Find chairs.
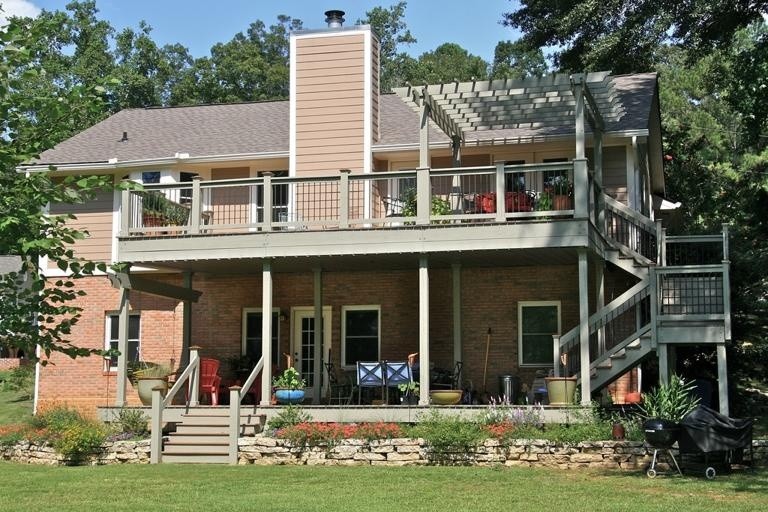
[324,361,464,404]
[184,356,223,405]
[381,196,413,230]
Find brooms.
[475,327,492,404]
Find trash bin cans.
[498,374,520,405]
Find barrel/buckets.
[201,357,221,390]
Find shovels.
[480,327,492,405]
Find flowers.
[543,175,574,200]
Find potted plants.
[271,367,307,405]
[141,191,189,237]
[133,361,173,407]
[630,372,703,448]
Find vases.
[552,195,572,210]
[544,376,577,405]
